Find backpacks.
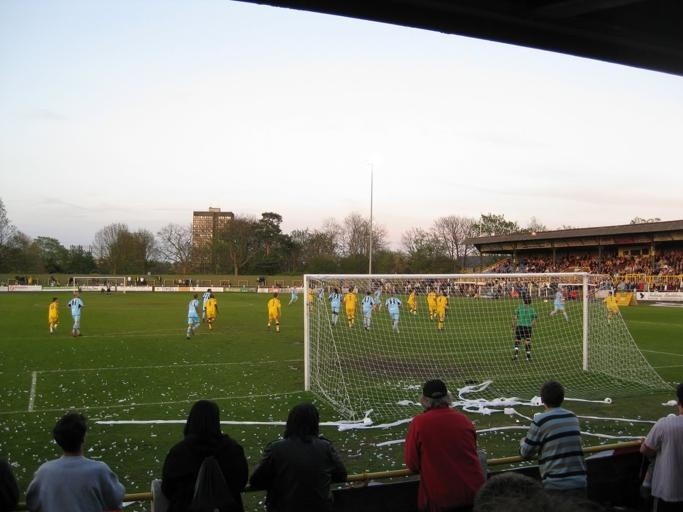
[188,436,231,512]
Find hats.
[423,380,447,399]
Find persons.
[250,402,347,512]
[265,293,281,333]
[25,414,127,512]
[204,294,218,330]
[492,249,683,292]
[0,459,19,512]
[548,288,568,321]
[640,382,683,511]
[519,381,588,498]
[202,289,214,322]
[0,275,284,297]
[403,379,487,512]
[161,399,248,512]
[552,494,606,512]
[48,297,60,334]
[604,291,619,326]
[185,294,201,339]
[291,277,451,335]
[473,472,555,512]
[66,292,86,337]
[512,295,538,363]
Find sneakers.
[510,354,532,361]
